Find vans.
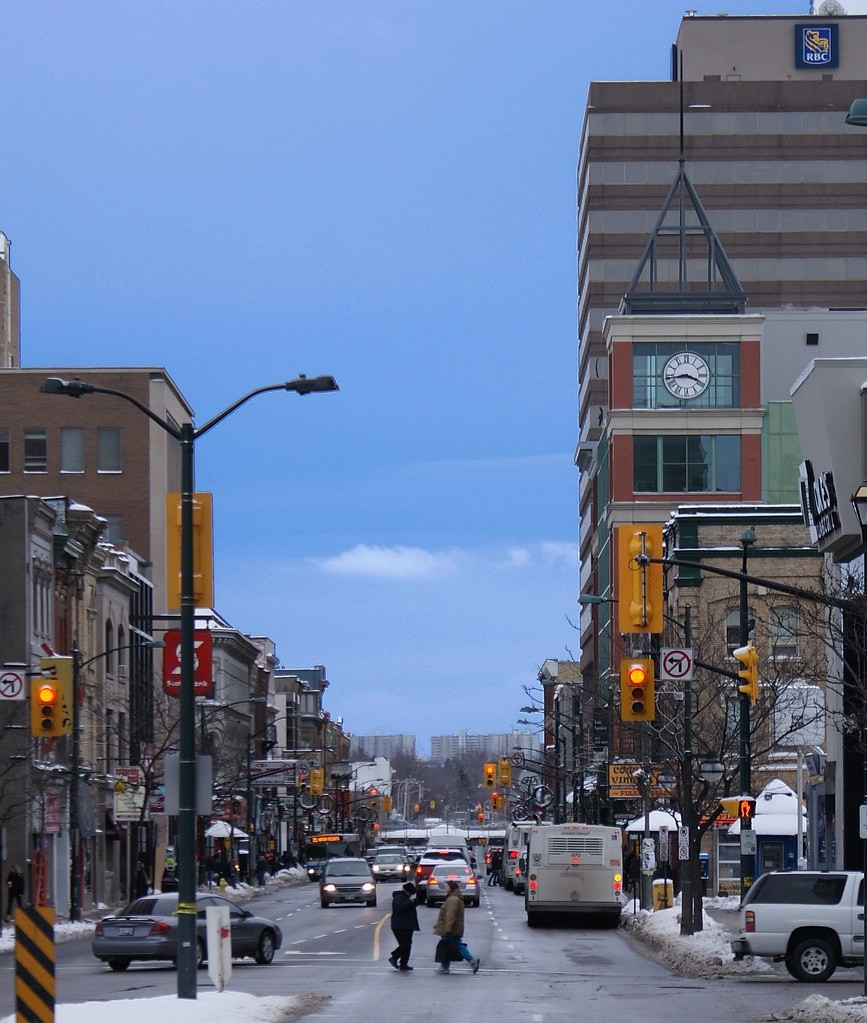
[483,822,553,893]
[731,869,865,982]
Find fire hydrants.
[219,878,227,893]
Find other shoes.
[628,886,632,893]
[389,957,399,969]
[493,884,497,886]
[399,964,413,971]
[471,958,480,974]
[437,966,450,975]
[488,884,492,886]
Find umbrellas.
[204,820,249,838]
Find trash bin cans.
[653,880,672,911]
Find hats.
[403,883,417,895]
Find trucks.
[519,824,623,928]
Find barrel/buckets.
[652,884,674,912]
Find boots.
[5,914,11,923]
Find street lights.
[520,706,585,823]
[336,764,377,835]
[513,745,561,826]
[312,759,353,836]
[293,745,338,862]
[247,714,302,884]
[517,719,567,825]
[198,697,265,886]
[578,594,725,935]
[540,679,623,825]
[354,778,383,852]
[70,641,165,920]
[361,778,430,830]
[39,373,340,999]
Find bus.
[302,834,358,883]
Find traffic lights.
[720,797,757,819]
[490,791,499,812]
[732,641,759,707]
[29,678,64,737]
[483,763,497,789]
[620,659,656,721]
[478,805,483,824]
[371,801,376,811]
[299,773,306,790]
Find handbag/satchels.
[435,940,467,962]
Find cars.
[415,837,483,908]
[316,858,378,907]
[92,892,282,971]
[364,846,426,883]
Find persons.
[388,882,421,971]
[433,880,481,973]
[4,863,25,924]
[622,847,640,894]
[256,850,297,886]
